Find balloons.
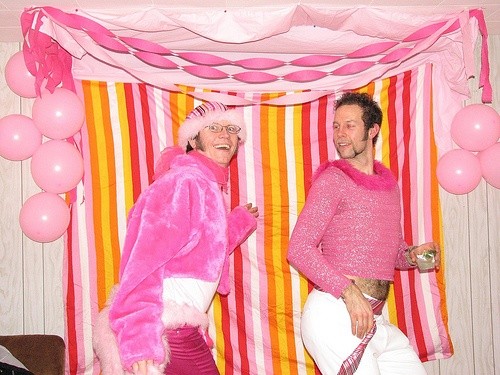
[449,104,500,152]
[30,139,84,194]
[19,193,70,243]
[5,50,53,99]
[0,114,42,161]
[437,149,482,195]
[32,88,85,140]
[478,142,500,189]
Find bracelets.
[406,246,419,266]
[341,279,355,299]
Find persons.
[287,93,442,375]
[93,102,259,375]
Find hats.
[178,101,247,150]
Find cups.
[414,249,435,274]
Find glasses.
[203,123,241,134]
